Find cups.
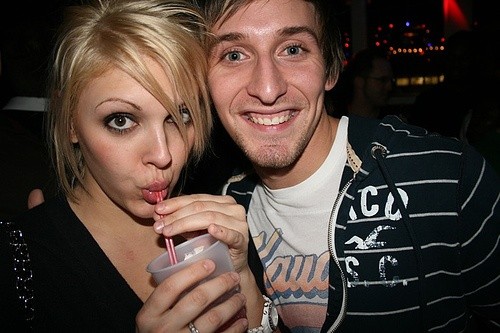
[145,234,241,312]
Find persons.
[28,0,500,333]
[0,0,280,333]
[340,51,395,118]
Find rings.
[186,321,203,333]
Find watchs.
[245,294,280,333]
